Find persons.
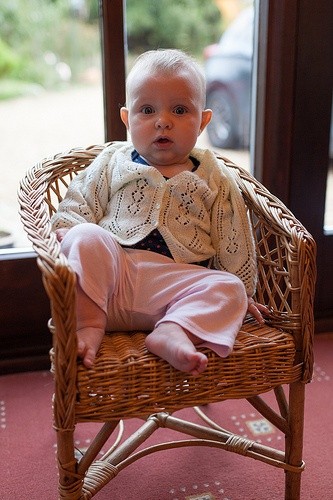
[50,50,271,376]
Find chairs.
[18,140,317,500]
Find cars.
[200,4,255,147]
